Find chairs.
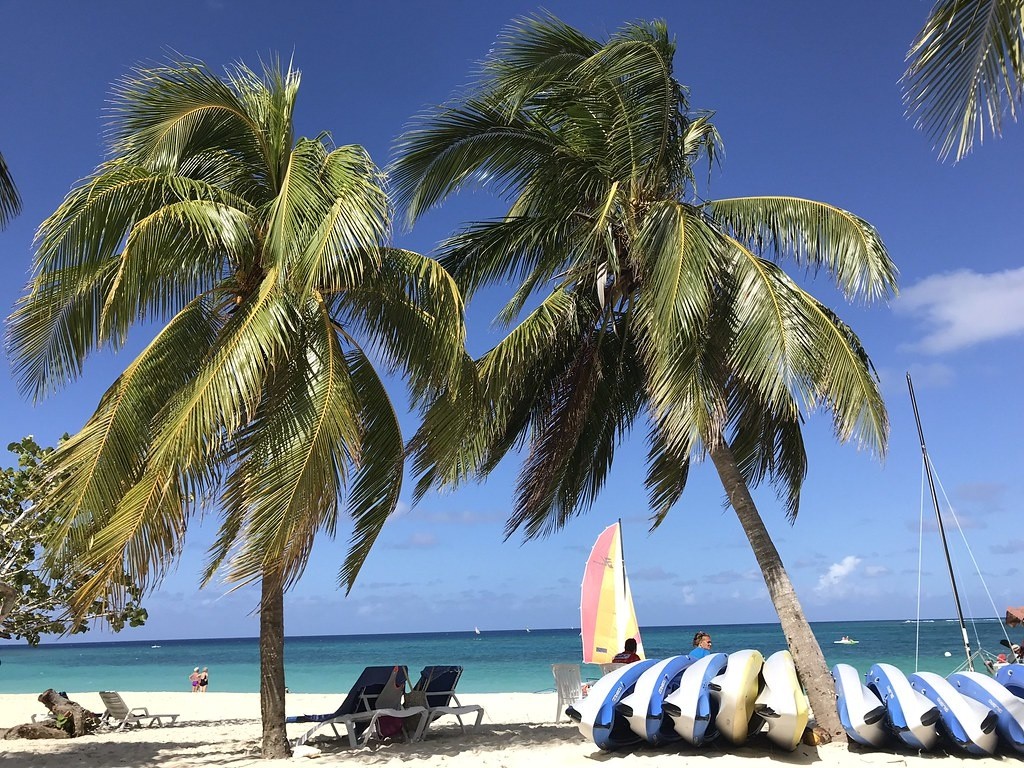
[551,664,585,723]
[89,692,180,732]
[598,662,628,676]
[286,666,484,751]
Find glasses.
[700,631,709,635]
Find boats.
[562,655,1024,758]
[151,645,161,648]
[833,640,861,645]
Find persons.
[197,667,209,692]
[689,631,711,658]
[842,636,849,641]
[189,667,200,692]
[1007,644,1024,664]
[612,638,641,663]
